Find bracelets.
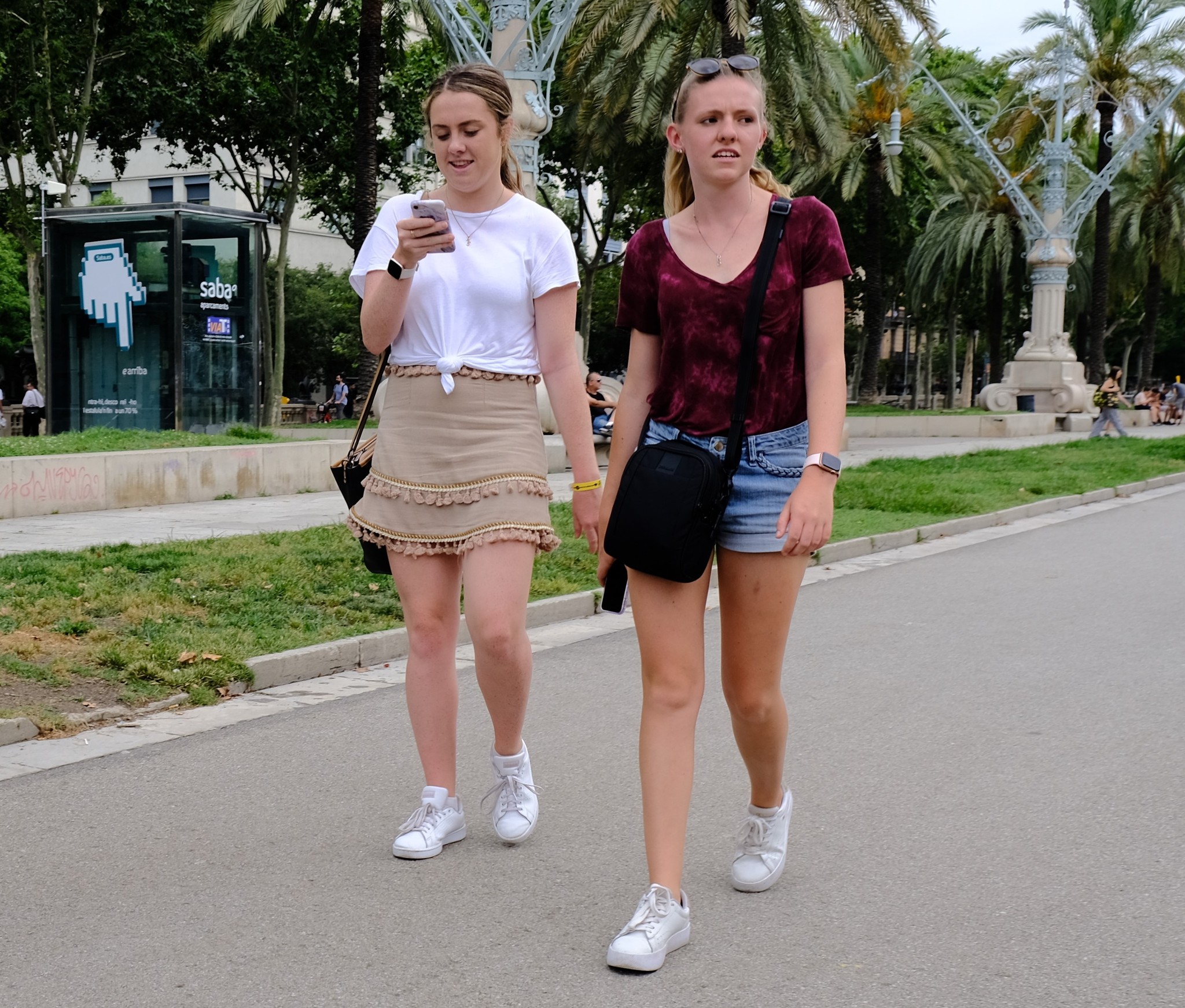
[569,479,601,492]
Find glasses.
[672,51,760,122]
[592,378,602,382]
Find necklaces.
[694,182,752,268]
[444,183,504,245]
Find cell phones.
[600,562,629,614]
[411,200,456,253]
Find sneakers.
[606,881,690,971]
[392,786,465,859]
[480,739,545,844]
[730,781,792,892]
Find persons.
[597,58,857,973]
[0,382,43,437]
[586,371,616,438]
[328,373,358,421]
[347,66,607,864]
[1087,366,1185,437]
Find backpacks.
[335,383,351,400]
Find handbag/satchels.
[26,409,41,424]
[330,344,392,574]
[603,440,728,584]
[1093,392,1120,409]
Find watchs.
[803,452,841,479]
[388,254,419,281]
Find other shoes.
[1152,418,1180,426]
[599,423,613,435]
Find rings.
[410,230,417,238]
